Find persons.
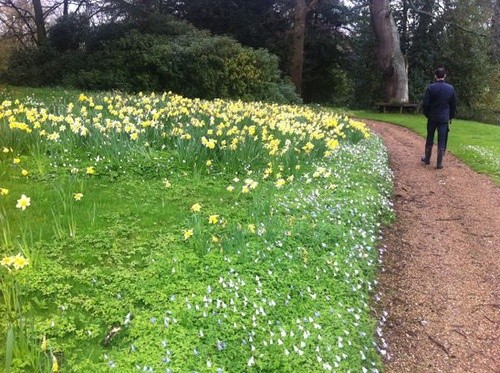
[419,66,458,168]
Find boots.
[420,144,433,165]
[436,145,443,168]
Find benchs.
[374,101,420,115]
